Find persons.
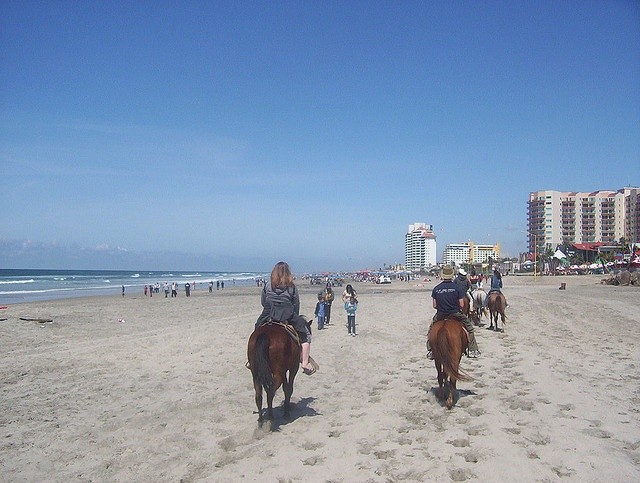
[313,294,327,330]
[163,282,170,298]
[171,282,178,297]
[320,281,334,325]
[184,282,191,297]
[208,280,213,293]
[450,268,474,314]
[219,273,417,291]
[192,280,196,289]
[482,269,510,310]
[424,263,482,359]
[468,269,480,291]
[343,296,357,337]
[342,284,359,303]
[142,280,160,297]
[253,259,315,372]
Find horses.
[427,318,478,409]
[486,290,509,330]
[471,288,488,322]
[247,315,313,422]
[462,295,469,317]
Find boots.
[564,282,566,289]
[559,282,564,289]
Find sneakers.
[352,332,356,336]
[426,349,433,359]
[468,349,481,357]
[348,332,351,335]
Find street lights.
[526,231,537,280]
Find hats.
[325,282,331,288]
[438,264,455,279]
[457,268,468,275]
